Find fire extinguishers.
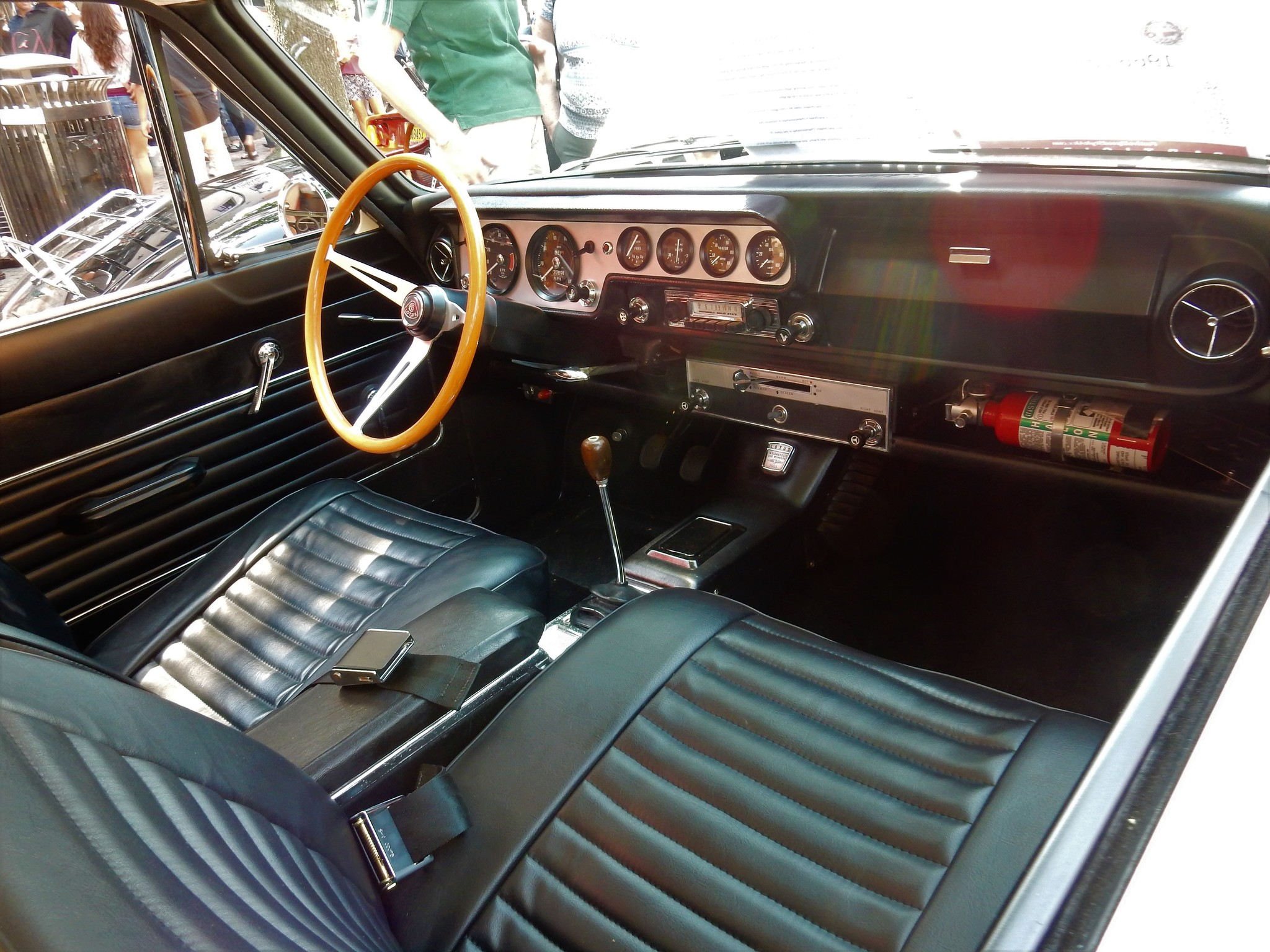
[942,380,1172,474]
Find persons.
[529,0,609,164]
[0,0,276,195]
[334,0,388,136]
[359,0,557,189]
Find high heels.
[244,144,259,161]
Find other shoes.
[227,141,243,152]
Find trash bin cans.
[0,74,144,266]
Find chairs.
[0,477,1107,952]
